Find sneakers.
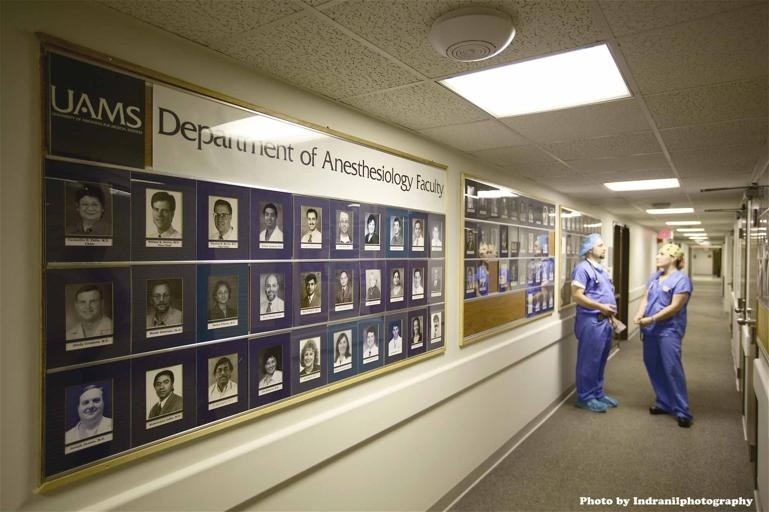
[574,396,608,413]
[596,394,619,407]
[649,405,673,413]
[676,409,691,427]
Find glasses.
[214,213,231,220]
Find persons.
[570,233,619,413]
[209,357,238,401]
[301,340,320,376]
[206,197,238,244]
[413,220,423,247]
[412,269,424,295]
[391,218,402,245]
[367,271,380,300]
[148,186,180,245]
[337,211,351,245]
[431,224,442,247]
[301,274,320,309]
[365,214,378,244]
[209,281,236,320]
[66,383,113,442]
[64,182,115,243]
[411,318,423,342]
[391,271,403,297]
[464,181,555,315]
[147,279,181,328]
[253,203,285,243]
[257,351,282,385]
[432,314,441,339]
[299,210,322,247]
[633,243,694,427]
[260,273,284,313]
[431,269,441,293]
[334,333,352,366]
[363,327,379,359]
[148,369,184,417]
[335,271,352,303]
[67,285,111,338]
[388,323,402,354]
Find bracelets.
[650,315,656,324]
